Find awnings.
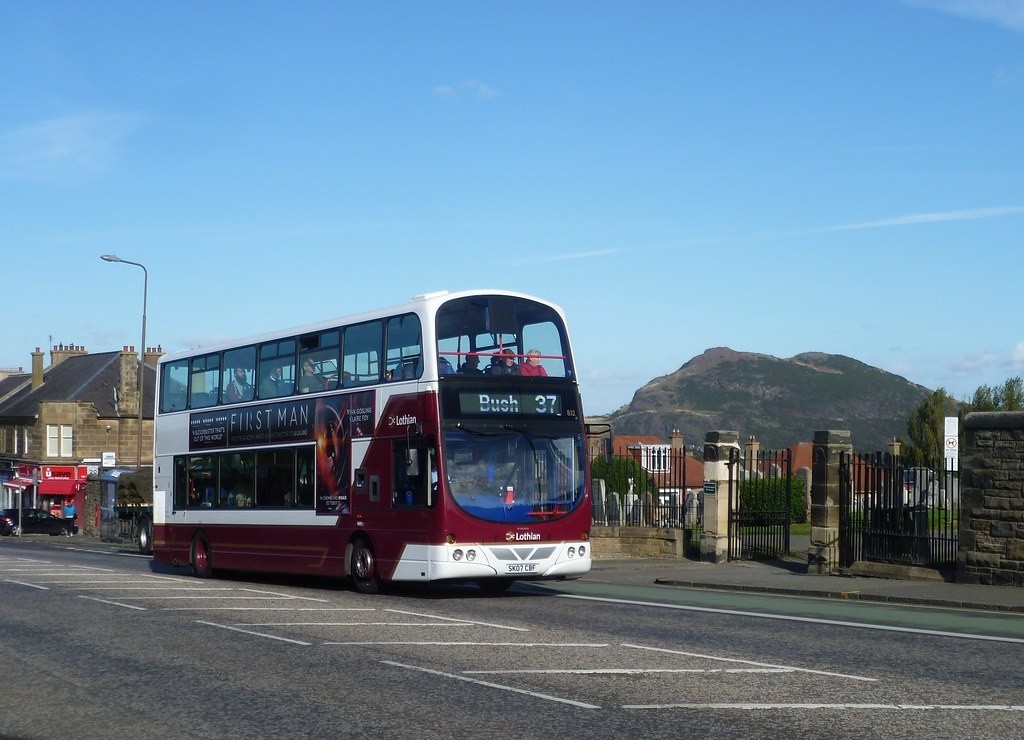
[2,477,39,492]
[39,478,77,495]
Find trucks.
[113,464,154,555]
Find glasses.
[304,364,317,367]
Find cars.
[0,508,79,538]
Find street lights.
[98,254,149,467]
[104,425,112,454]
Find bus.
[151,292,612,594]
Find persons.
[64,498,77,537]
[176,349,550,510]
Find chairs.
[193,477,228,507]
[183,372,356,406]
[439,359,491,375]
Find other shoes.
[67,535,69,538]
[70,533,73,538]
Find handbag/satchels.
[73,514,78,520]
